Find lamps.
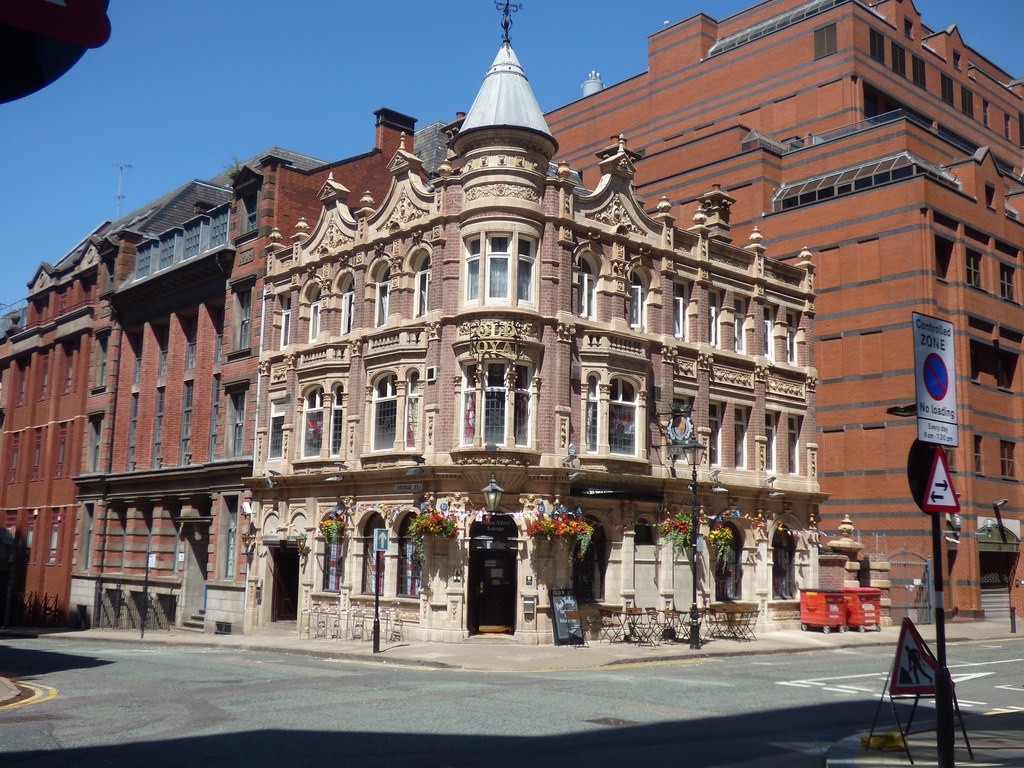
[406,468,423,475]
[325,476,343,481]
[769,493,785,498]
[334,462,348,472]
[708,470,721,482]
[942,516,965,536]
[993,498,1009,507]
[974,519,996,538]
[411,455,426,466]
[268,470,281,477]
[567,472,586,481]
[562,455,577,468]
[264,478,277,489]
[712,485,728,493]
[481,474,505,513]
[764,477,776,488]
[240,532,254,560]
[296,534,308,556]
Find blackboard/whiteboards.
[548,588,584,646]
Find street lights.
[681,430,708,651]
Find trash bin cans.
[837,587,881,633]
[798,588,850,634]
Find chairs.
[564,603,760,646]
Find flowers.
[707,523,733,575]
[408,509,459,567]
[525,512,594,569]
[659,510,693,564]
[319,519,347,546]
